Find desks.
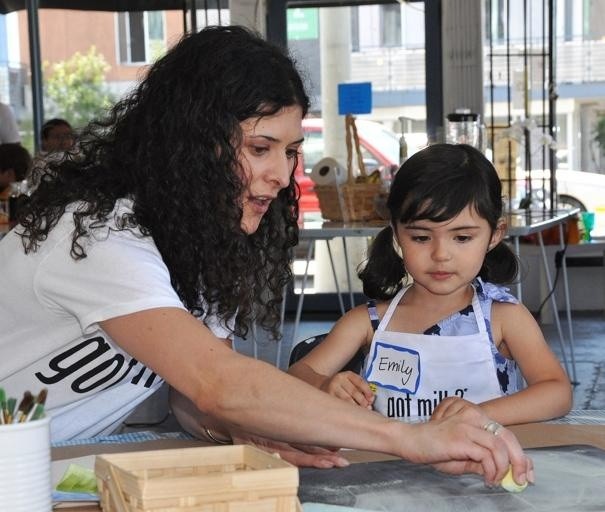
[278,206,582,392]
[50,406,605,511]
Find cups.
[0,410,52,512]
[446,112,487,157]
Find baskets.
[314,112,384,222]
[95,444,300,511]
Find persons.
[0,24,535,489]
[31,118,78,185]
[288,141,573,426]
[0,103,20,145]
[0,144,30,232]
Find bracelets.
[203,418,231,445]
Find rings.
[484,421,504,435]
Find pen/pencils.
[0,386,47,424]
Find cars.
[291,118,420,213]
